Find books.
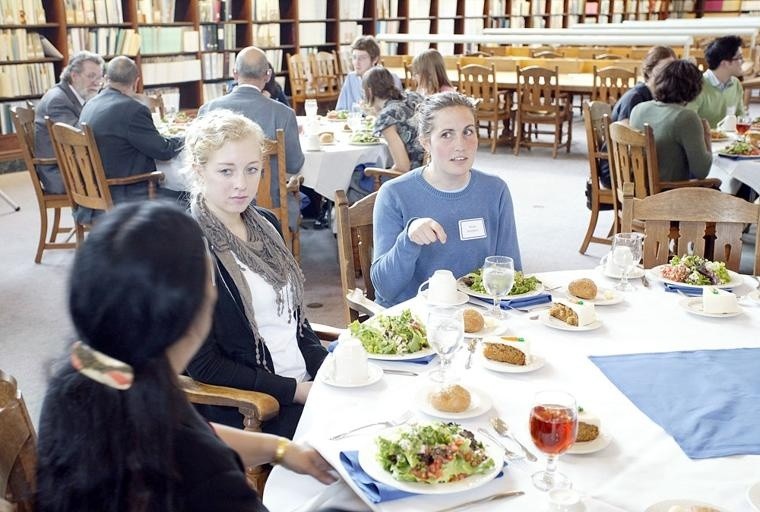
[64,0,329,117]
[338,1,679,56]
[0,0,57,135]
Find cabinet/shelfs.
[298,0,338,98]
[338,1,510,73]
[252,0,298,108]
[508,0,695,27]
[0,0,70,161]
[695,0,760,18]
[130,0,203,120]
[196,0,253,111]
[61,0,141,96]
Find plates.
[417,387,492,419]
[566,289,624,304]
[687,299,747,319]
[350,139,383,145]
[344,129,352,132]
[359,426,506,493]
[456,275,545,300]
[566,412,611,455]
[365,342,437,360]
[320,362,382,385]
[651,265,742,289]
[596,266,645,280]
[539,310,602,333]
[326,115,351,121]
[320,142,336,145]
[416,292,472,308]
[482,355,546,374]
[710,118,760,158]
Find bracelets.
[269,435,289,467]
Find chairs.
[0,370,39,512]
[166,310,348,504]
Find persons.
[33,49,106,197]
[27,198,339,512]
[597,34,758,262]
[410,48,456,99]
[173,107,327,472]
[345,65,426,203]
[71,55,191,231]
[369,90,525,311]
[333,34,405,115]
[194,44,306,236]
[226,57,292,108]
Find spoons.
[489,414,539,463]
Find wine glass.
[482,256,513,324]
[304,100,318,128]
[612,232,642,293]
[162,104,177,127]
[425,303,465,385]
[530,392,578,493]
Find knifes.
[437,492,525,510]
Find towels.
[339,450,507,503]
[325,340,437,365]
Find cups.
[304,134,322,149]
[419,268,457,303]
[331,338,367,379]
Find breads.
[430,382,472,414]
[567,277,598,302]
[549,299,581,329]
[460,307,485,333]
[574,421,599,443]
[482,339,526,368]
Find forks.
[478,428,527,466]
[330,410,413,441]
